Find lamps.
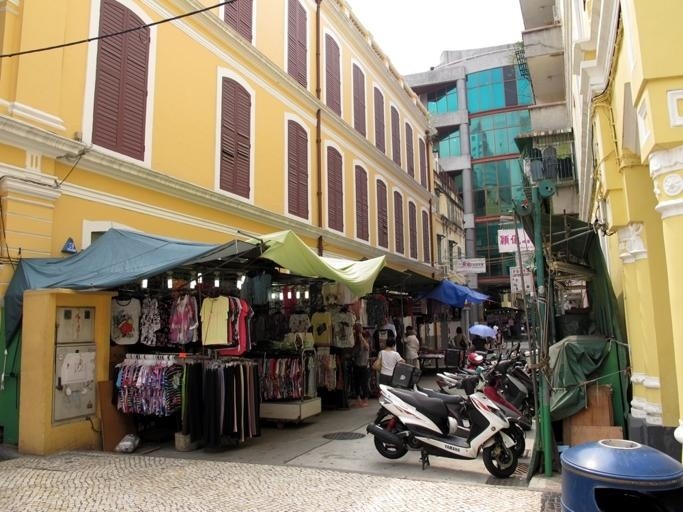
[592,198,608,237]
[140,271,247,290]
[271,283,311,301]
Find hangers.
[114,353,240,369]
[255,307,353,315]
[120,289,248,298]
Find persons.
[494,329,502,349]
[400,327,420,370]
[472,334,487,352]
[372,334,407,398]
[348,328,372,409]
[452,326,469,361]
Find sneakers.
[350,399,370,408]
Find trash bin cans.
[560,439,683,512]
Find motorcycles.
[366,341,539,479]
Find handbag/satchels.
[460,339,471,348]
[371,358,382,371]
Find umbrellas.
[468,323,496,340]
